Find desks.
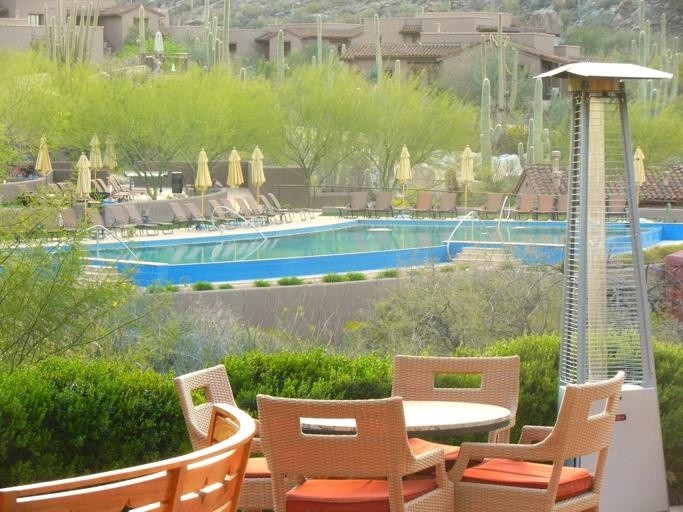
[247,400,512,450]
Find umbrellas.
[633,146,646,207]
[154,29,164,52]
[89,133,103,192]
[251,145,266,204]
[396,144,412,215]
[461,145,474,213]
[104,135,118,193]
[195,148,213,216]
[74,152,91,224]
[227,147,244,211]
[35,133,52,193]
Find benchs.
[0,399,258,511]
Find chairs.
[256,391,454,512]
[474,193,504,220]
[172,364,293,510]
[407,192,434,219]
[512,194,538,220]
[389,352,521,461]
[1,172,301,259]
[338,192,369,219]
[363,192,393,219]
[605,195,628,222]
[430,192,458,219]
[533,194,556,219]
[554,195,567,220]
[440,368,627,512]
